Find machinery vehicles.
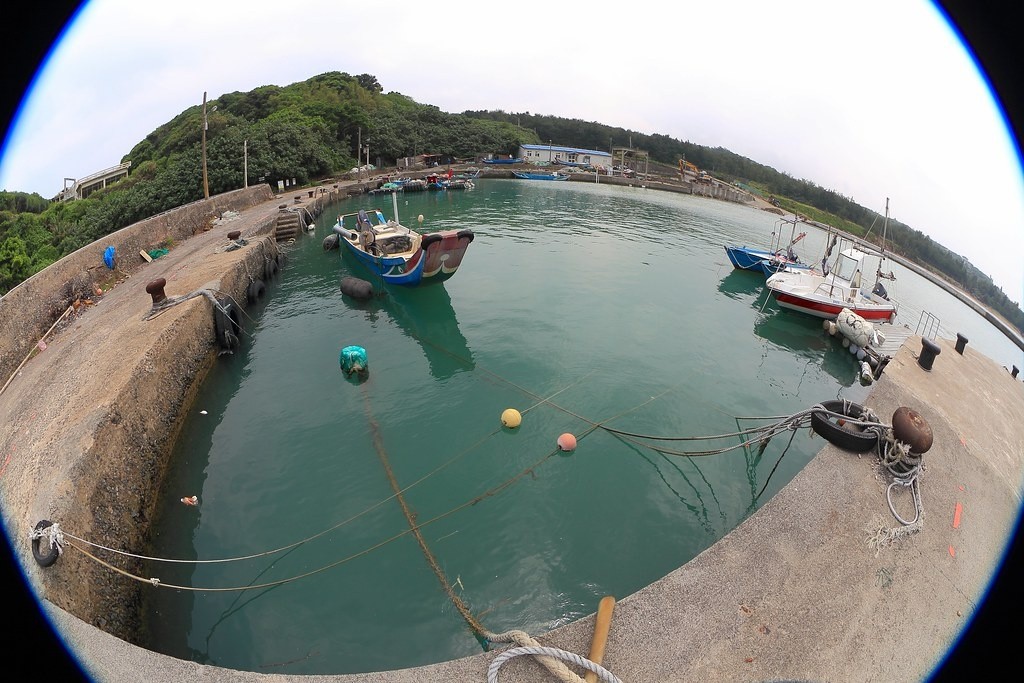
[679,158,713,186]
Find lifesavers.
[810,397,885,454]
[247,253,284,305]
[30,519,62,568]
[212,298,246,355]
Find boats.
[404,178,476,190]
[330,208,474,289]
[511,169,572,181]
[724,197,900,325]
